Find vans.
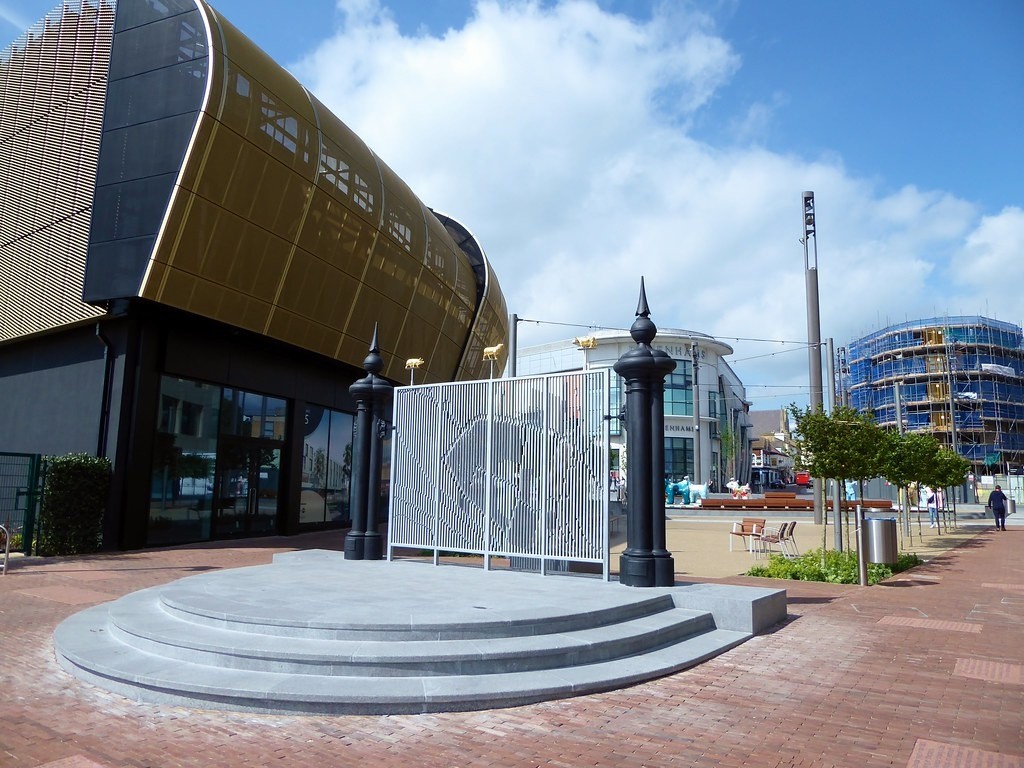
[177,452,247,504]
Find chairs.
[765,522,800,558]
[608,511,620,535]
[730,517,767,555]
[754,522,790,560]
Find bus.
[796,472,810,486]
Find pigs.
[405,356,424,369]
[572,336,597,348]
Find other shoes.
[930,524,935,528]
[1001,528,1007,531]
[936,524,939,527]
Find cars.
[770,479,786,489]
[806,480,813,488]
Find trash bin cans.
[861,517,898,564]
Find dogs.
[482,343,505,360]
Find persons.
[926,488,940,528]
[988,485,1008,531]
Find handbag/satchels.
[928,494,934,503]
